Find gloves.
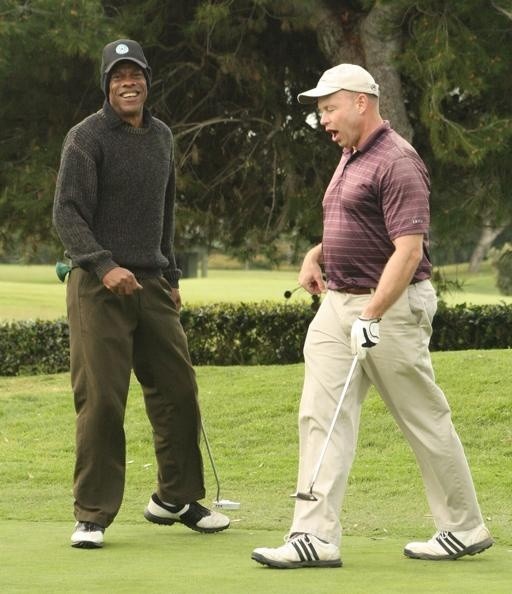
[350,316,381,361]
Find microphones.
[283,284,303,299]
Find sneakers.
[144,494,230,533]
[403,523,492,561]
[70,520,105,549]
[251,533,343,569]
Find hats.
[297,64,380,106]
[102,39,148,75]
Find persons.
[252,63,492,570]
[52,39,231,549]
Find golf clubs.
[200,419,240,508]
[290,356,358,502]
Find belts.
[342,284,377,295]
